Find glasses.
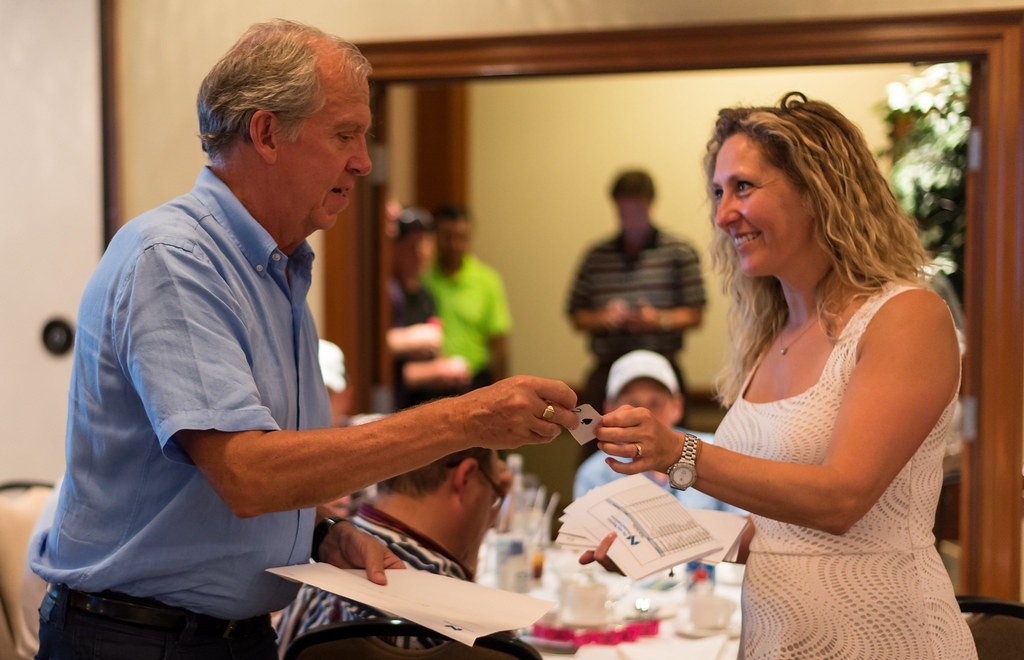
[444,456,508,511]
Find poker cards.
[563,402,608,448]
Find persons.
[275,380,514,660]
[564,170,705,458]
[387,201,512,413]
[28,18,580,660]
[573,92,979,660]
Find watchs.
[666,433,698,491]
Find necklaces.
[779,315,820,357]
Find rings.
[635,444,643,459]
[542,405,555,418]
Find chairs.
[0,483,57,659]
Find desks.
[479,543,747,660]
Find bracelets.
[309,517,351,559]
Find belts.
[49,584,272,641]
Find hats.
[606,350,679,403]
